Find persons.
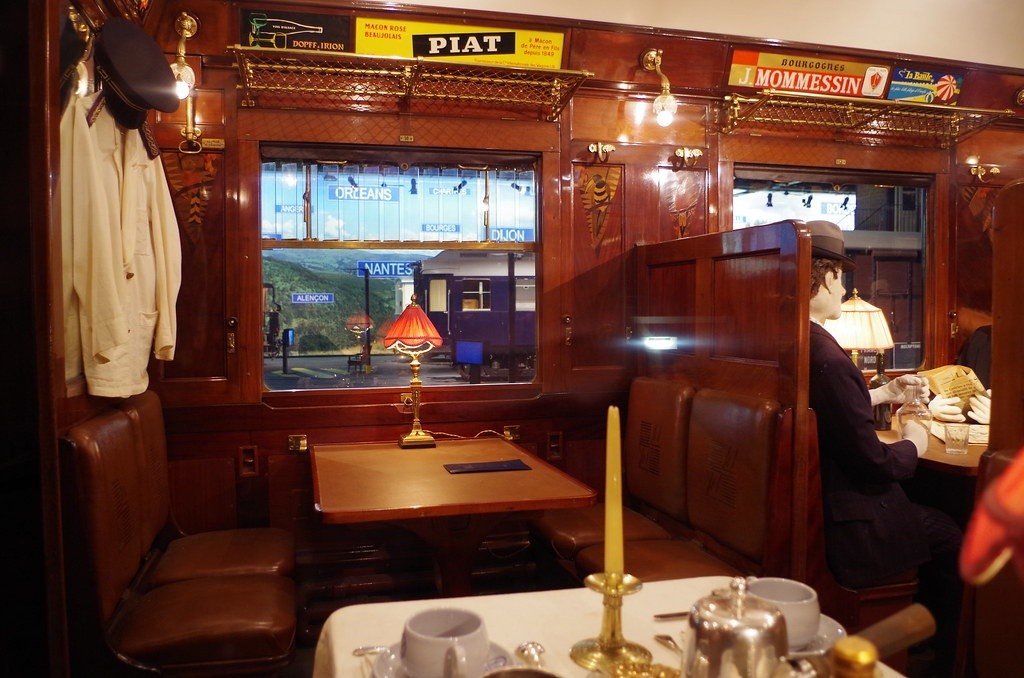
[928,324,992,425]
[806,220,965,669]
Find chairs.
[576,387,794,588]
[118,387,298,591]
[54,408,297,678]
[527,376,698,593]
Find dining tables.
[871,413,990,662]
[312,576,905,678]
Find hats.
[93,20,182,129]
[804,220,858,273]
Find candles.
[604,405,624,582]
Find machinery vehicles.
[263,283,281,360]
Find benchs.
[953,445,1024,677]
[810,408,918,588]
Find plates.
[373,640,516,678]
[787,613,848,660]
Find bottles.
[895,375,933,440]
[869,353,893,431]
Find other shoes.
[907,639,934,660]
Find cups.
[399,606,490,678]
[748,577,821,652]
[944,423,970,454]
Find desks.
[309,434,599,601]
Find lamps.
[823,287,894,368]
[169,10,201,100]
[383,294,443,448]
[639,48,678,127]
